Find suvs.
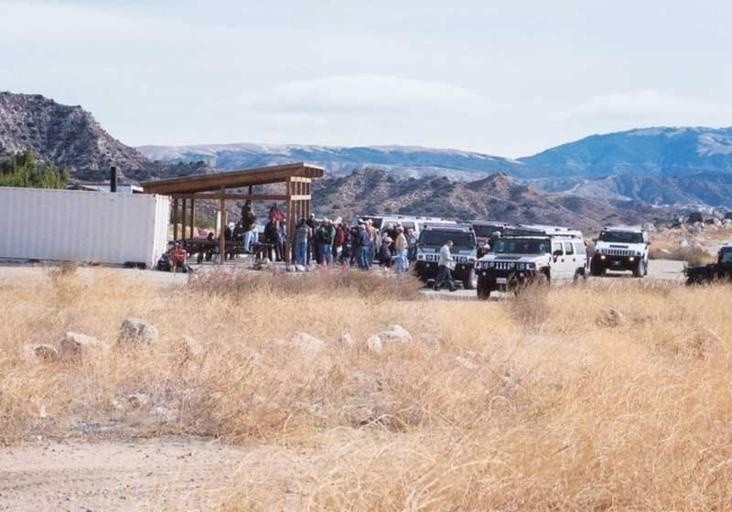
[473,234,588,300]
[716,243,731,265]
[590,224,650,278]
[414,224,479,289]
[349,214,584,262]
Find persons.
[169,240,187,278]
[474,228,501,256]
[432,239,459,292]
[196,199,430,274]
